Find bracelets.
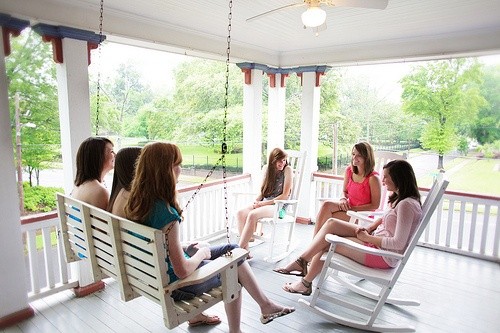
[339,197,347,201]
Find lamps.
[244,0,388,30]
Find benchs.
[56,193,248,329]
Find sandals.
[282,278,312,296]
[260,306,295,324]
[273,257,307,277]
[187,313,221,326]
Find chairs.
[317,150,408,225]
[232,146,310,263]
[297,170,451,333]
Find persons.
[273,160,423,296]
[67,137,117,261]
[123,142,296,324]
[307,142,381,266]
[106,146,221,327]
[237,147,293,259]
